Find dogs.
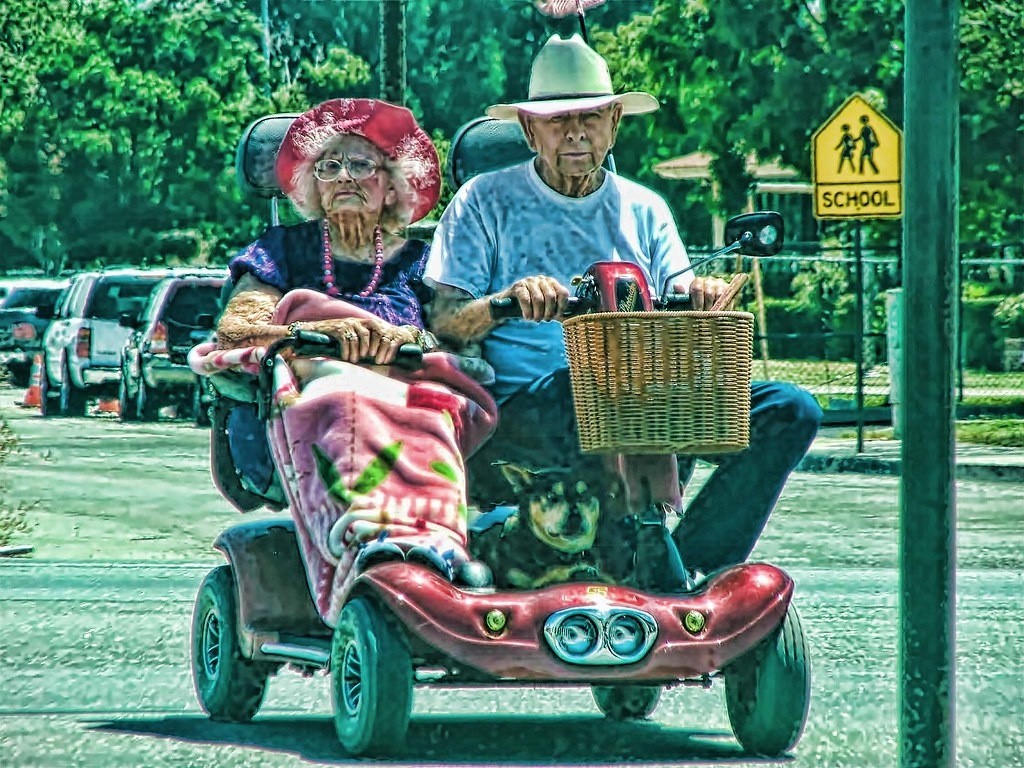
[466,453,617,591]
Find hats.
[485,34,659,121]
[276,98,441,225]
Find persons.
[421,33,824,591]
[218,99,497,590]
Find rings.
[344,333,358,341]
[381,336,391,343]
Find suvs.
[0,270,88,387]
[34,265,186,418]
[117,267,233,426]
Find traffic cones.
[94,398,120,417]
[18,354,44,410]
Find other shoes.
[408,548,452,579]
[355,542,404,574]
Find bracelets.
[288,320,306,334]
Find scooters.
[186,110,815,759]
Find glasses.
[312,158,386,183]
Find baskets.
[563,310,754,455]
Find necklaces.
[323,218,384,297]
